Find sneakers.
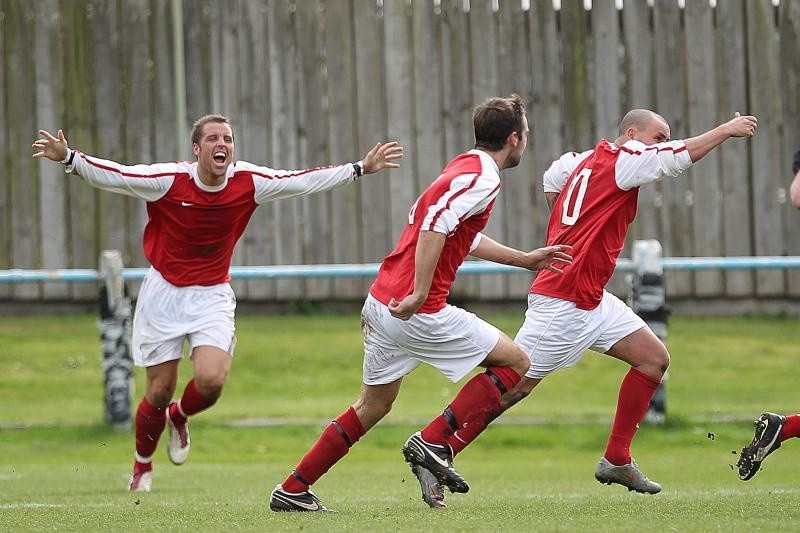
[268,484,327,511]
[166,401,193,464]
[735,412,786,482]
[125,462,151,494]
[405,443,454,508]
[593,456,662,494]
[402,431,470,493]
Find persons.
[29,113,404,492]
[790,150,800,211]
[735,412,800,480]
[266,93,577,512]
[412,108,760,509]
